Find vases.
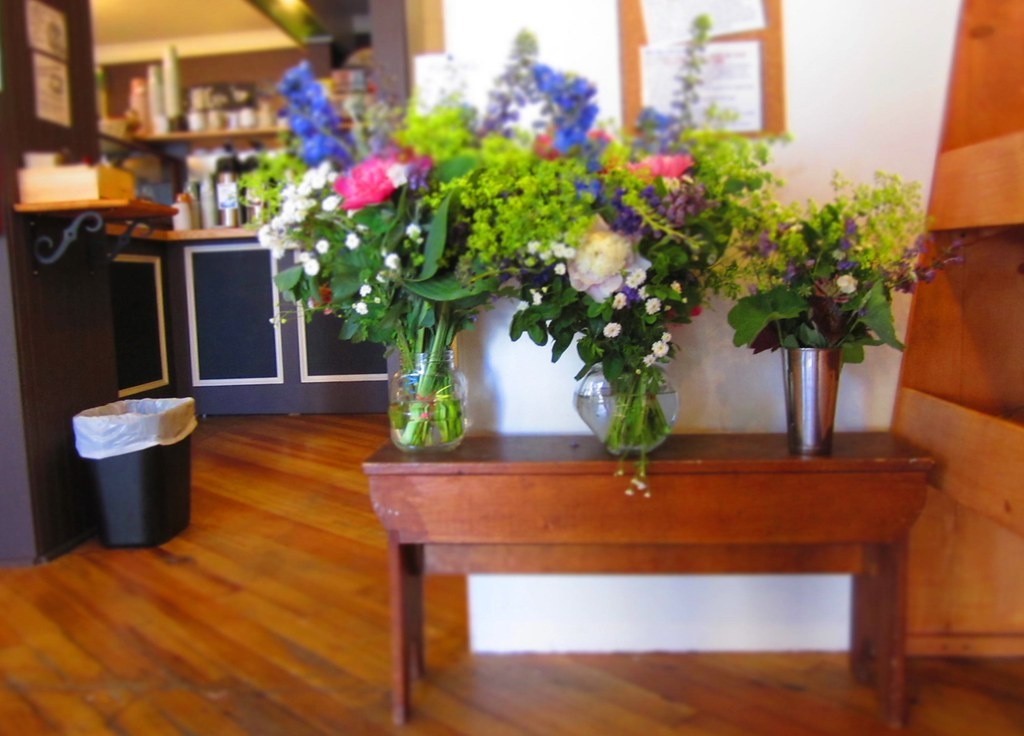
[571,357,678,453]
[386,347,469,450]
[772,333,848,447]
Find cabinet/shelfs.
[100,95,398,419]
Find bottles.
[169,157,270,229]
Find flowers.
[235,12,1008,502]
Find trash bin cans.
[73,397,198,549]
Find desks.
[363,435,942,728]
